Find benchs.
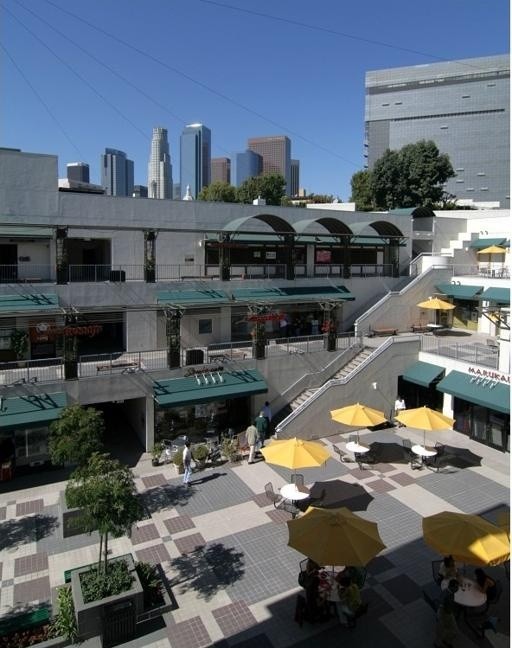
[180,275,214,280]
[410,325,432,333]
[209,351,247,363]
[94,363,136,375]
[369,328,400,338]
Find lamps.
[469,374,500,390]
[185,363,226,386]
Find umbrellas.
[416,297,456,326]
[330,402,385,446]
[286,505,387,582]
[258,436,331,487]
[476,245,506,277]
[393,405,457,449]
[422,511,510,577]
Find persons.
[182,441,197,487]
[254,411,267,448]
[474,568,496,600]
[298,558,367,630]
[246,420,258,464]
[261,401,271,438]
[435,557,456,583]
[434,579,459,648]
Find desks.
[426,324,443,336]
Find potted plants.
[172,447,185,474]
[150,442,164,467]
[191,445,209,467]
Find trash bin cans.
[186,350,204,365]
[109,271,125,282]
[99,598,136,648]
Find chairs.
[400,437,447,472]
[332,434,385,471]
[291,556,510,648]
[162,429,241,464]
[475,262,507,278]
[264,474,326,521]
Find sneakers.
[442,638,454,647]
[433,640,443,647]
